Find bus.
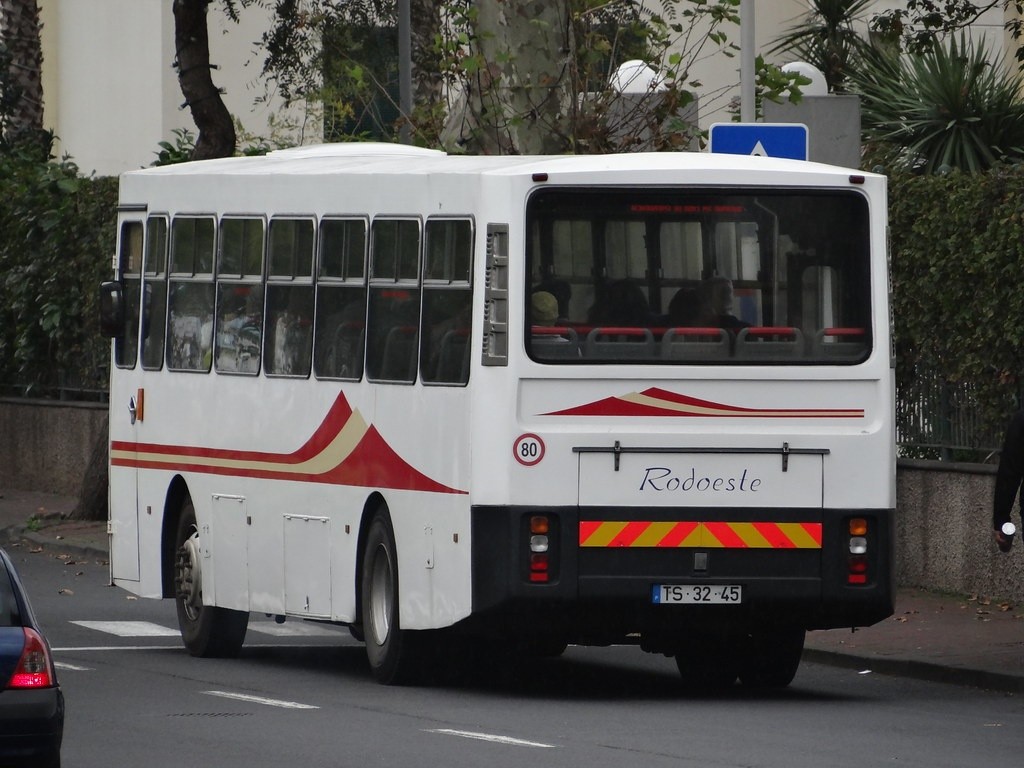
[106,142,900,690]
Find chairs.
[331,321,363,379]
[434,329,468,384]
[280,319,311,374]
[734,328,804,359]
[659,328,730,360]
[585,329,655,359]
[380,325,417,380]
[532,326,579,361]
[811,329,869,358]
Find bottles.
[999,522,1016,553]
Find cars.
[0,549,66,767]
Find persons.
[531,276,757,358]
[993,411,1024,546]
